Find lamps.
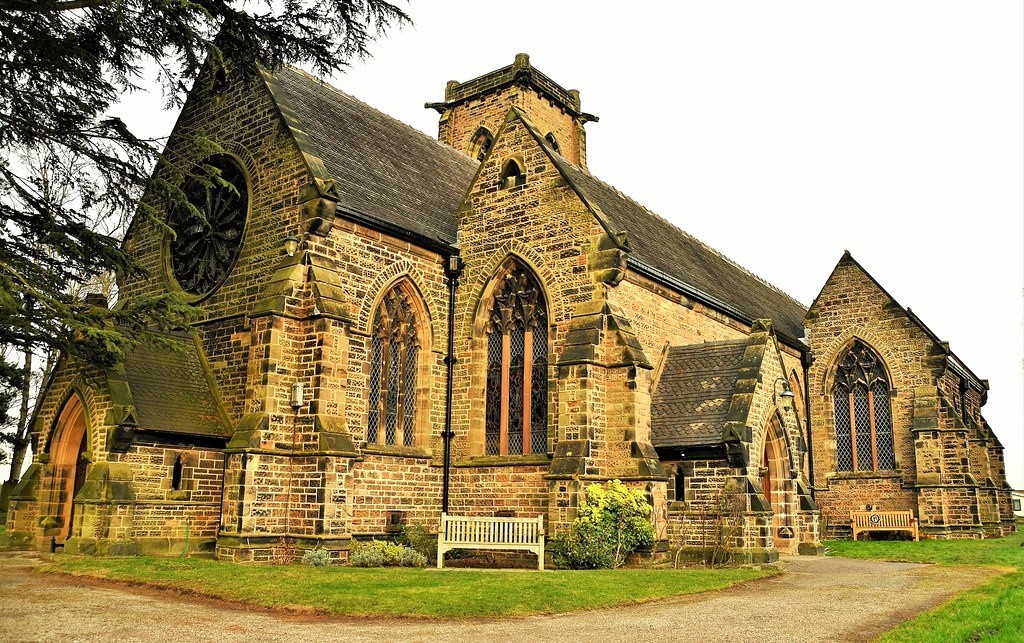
[773,377,795,413]
[284,224,304,258]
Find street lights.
[440,255,467,517]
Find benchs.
[437,512,545,571]
[850,508,918,542]
